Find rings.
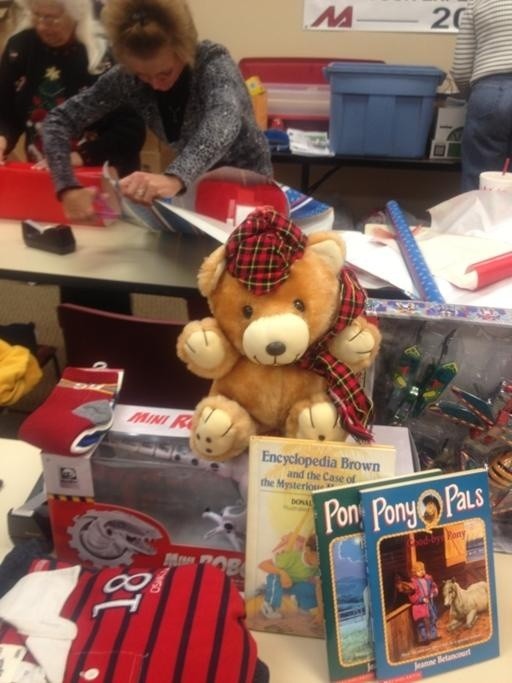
[136,187,148,196]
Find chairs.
[56,302,213,410]
[0,344,60,421]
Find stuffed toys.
[172,208,386,455]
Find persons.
[447,0,511,200]
[0,0,149,178]
[41,0,283,223]
[406,561,442,644]
[257,535,321,619]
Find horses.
[441,575,488,632]
[383,570,418,616]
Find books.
[311,469,447,682]
[241,431,397,641]
[356,469,504,682]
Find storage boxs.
[428,101,466,160]
[322,61,447,160]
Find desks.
[271,154,462,196]
[0,219,220,319]
